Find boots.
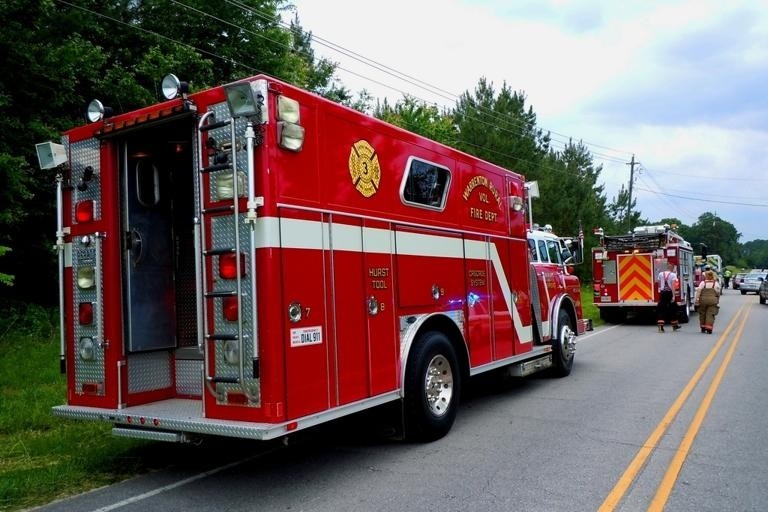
[700,323,712,334]
[657,320,665,332]
[671,320,681,331]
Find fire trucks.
[34,72,593,447]
[591,224,707,323]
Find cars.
[733,269,768,304]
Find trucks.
[693,254,724,295]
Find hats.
[704,265,711,269]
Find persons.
[657,265,681,333]
[702,264,720,281]
[694,271,720,334]
[724,268,732,289]
